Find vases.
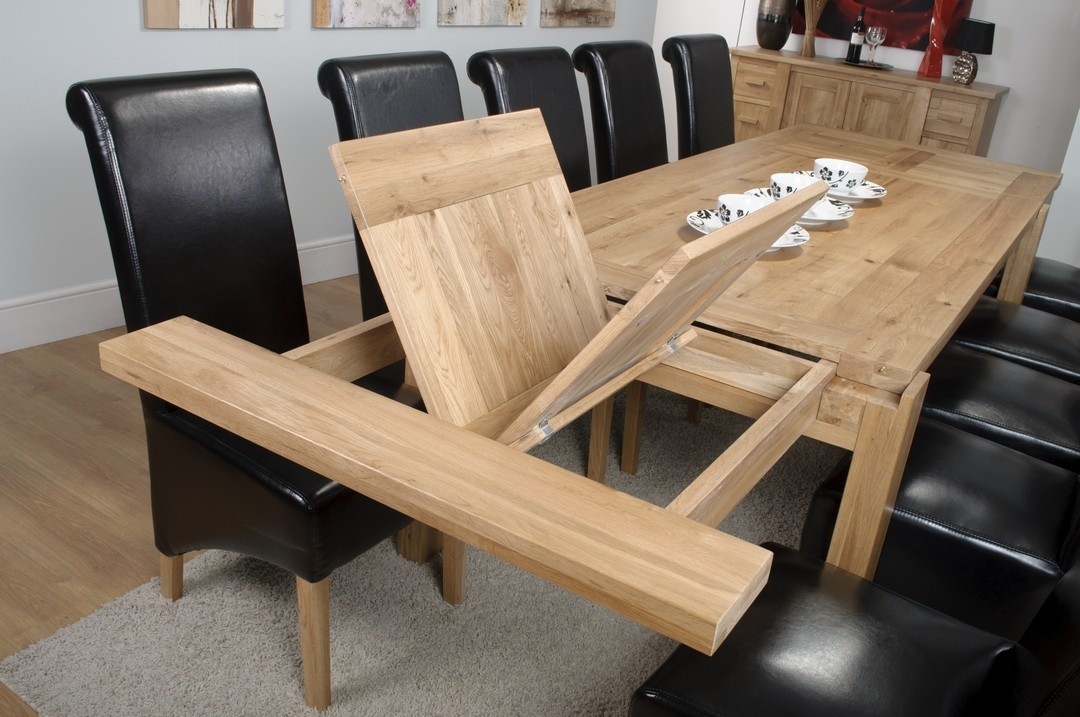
[918,0,959,77]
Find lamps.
[948,17,998,84]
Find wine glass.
[863,26,888,66]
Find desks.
[96,122,1063,656]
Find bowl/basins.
[814,158,869,193]
[717,193,771,226]
[770,173,828,217]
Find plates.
[791,171,887,203]
[837,58,894,69]
[687,209,810,251]
[743,187,856,225]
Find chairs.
[65,33,1080,717]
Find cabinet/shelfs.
[727,44,1012,156]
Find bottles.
[846,7,866,63]
